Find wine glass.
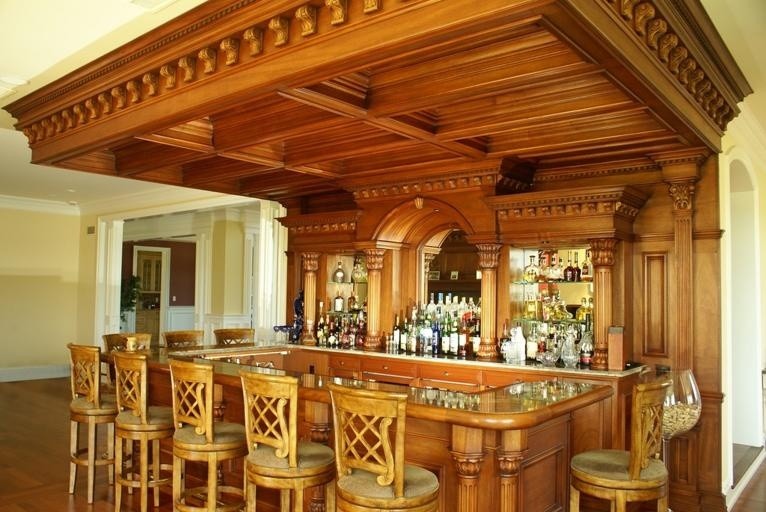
[636,368,701,512]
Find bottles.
[499,294,594,366]
[393,293,481,357]
[316,261,367,350]
[524,251,593,283]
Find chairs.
[569,376,671,511]
[167,358,248,512]
[214,328,255,344]
[103,331,152,387]
[324,380,440,512]
[68,343,125,504]
[112,351,186,511]
[238,371,337,512]
[162,330,205,348]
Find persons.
[293,288,304,341]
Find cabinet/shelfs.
[321,278,368,320]
[527,276,595,329]
[283,347,612,391]
[136,251,162,345]
